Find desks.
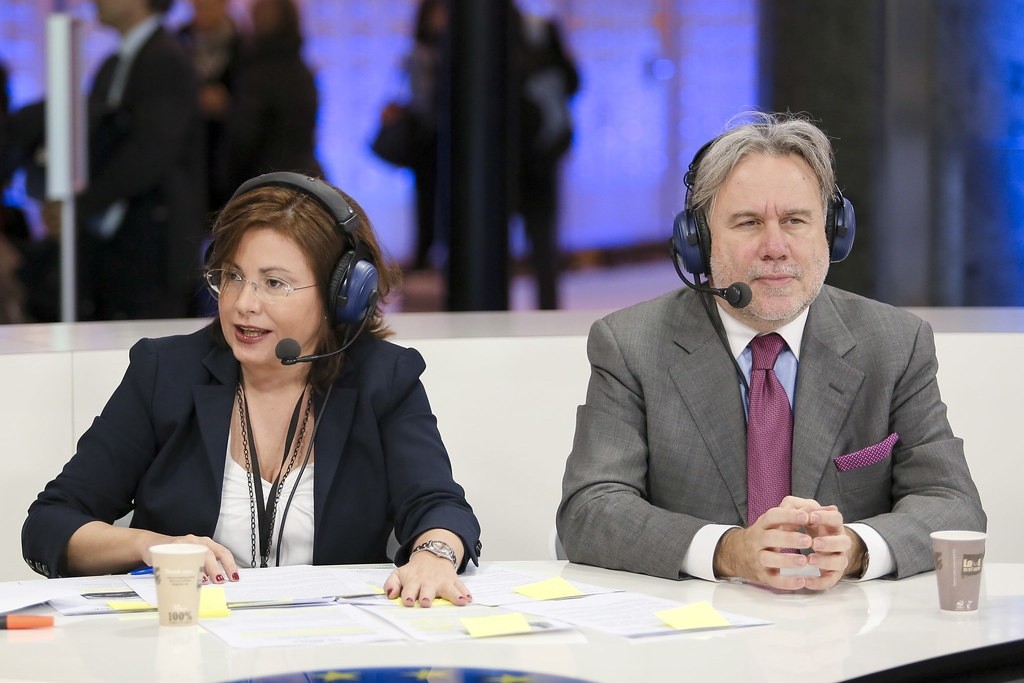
[1,558,1024,683]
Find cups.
[148,544,208,629]
[930,530,987,616]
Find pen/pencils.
[131,568,153,575]
[0,614,64,630]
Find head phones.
[201,172,380,327]
[667,122,856,276]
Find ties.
[748,332,801,590]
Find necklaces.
[237,385,313,568]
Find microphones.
[671,257,753,309]
[275,315,368,365]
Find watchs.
[410,540,457,571]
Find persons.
[555,111,987,591]
[21,172,482,607]
[370,0,584,310]
[0,0,327,324]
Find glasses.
[204,269,316,304]
